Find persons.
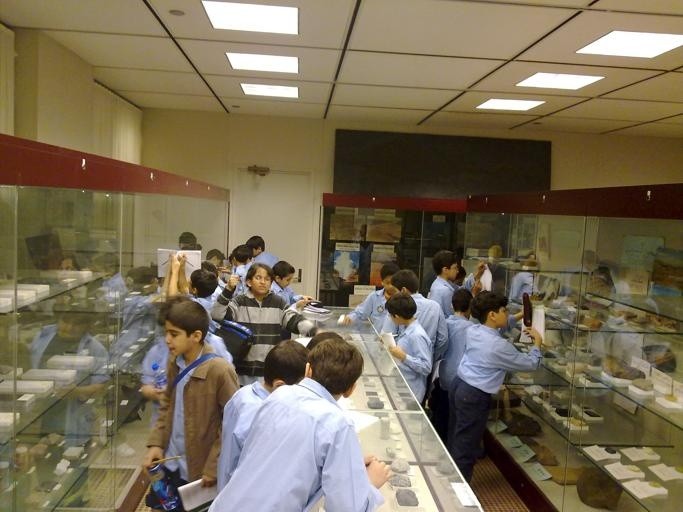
[166,234,341,386]
[143,294,233,434]
[217,340,309,495]
[31,251,155,507]
[141,301,239,511]
[339,262,400,338]
[428,245,540,483]
[380,293,433,404]
[551,248,661,402]
[207,338,390,511]
[391,269,449,408]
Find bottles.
[0,460,10,490]
[150,362,166,388]
[14,448,27,470]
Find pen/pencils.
[161,259,171,267]
[238,275,242,278]
[151,455,181,464]
[185,259,195,269]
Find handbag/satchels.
[215,319,254,366]
